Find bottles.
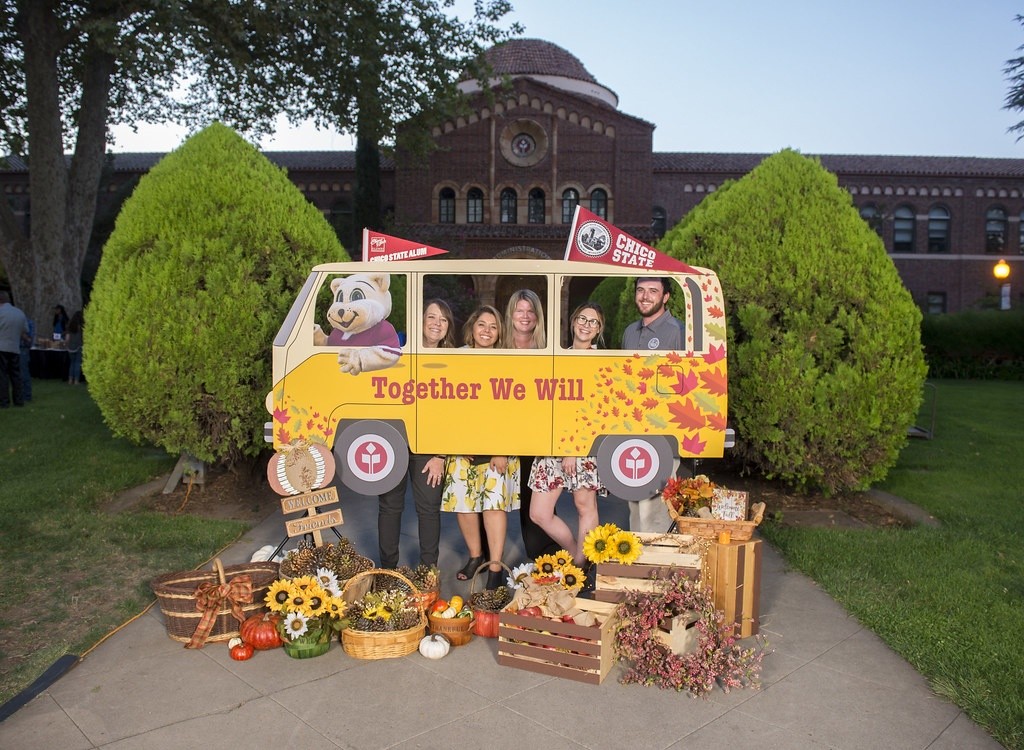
[36,338,60,350]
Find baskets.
[468,560,517,639]
[277,550,375,591]
[427,604,476,646]
[152,557,280,649]
[341,568,428,660]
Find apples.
[511,606,600,658]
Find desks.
[29,346,85,379]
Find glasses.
[575,315,601,328]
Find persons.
[440,305,522,591]
[622,276,681,536]
[527,304,609,591]
[378,299,455,571]
[505,288,558,561]
[0,291,84,409]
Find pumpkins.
[228,611,285,661]
[431,595,473,619]
[249,544,299,564]
[419,633,450,659]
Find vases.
[283,611,333,658]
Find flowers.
[265,475,776,701]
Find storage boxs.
[498,598,619,685]
[594,529,704,611]
[703,538,760,640]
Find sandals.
[486,565,503,591]
[456,553,487,581]
